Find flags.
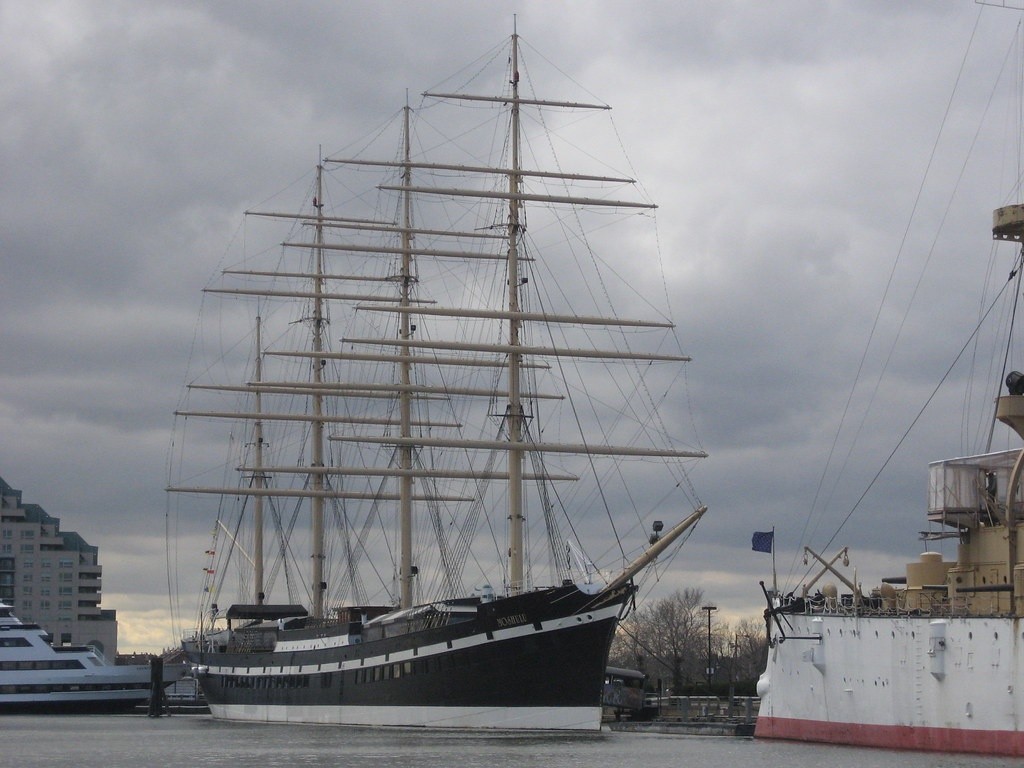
[751,531,774,553]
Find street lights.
[701,606,717,697]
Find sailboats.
[161,15,709,733]
[751,0,1024,757]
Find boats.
[0,598,162,716]
